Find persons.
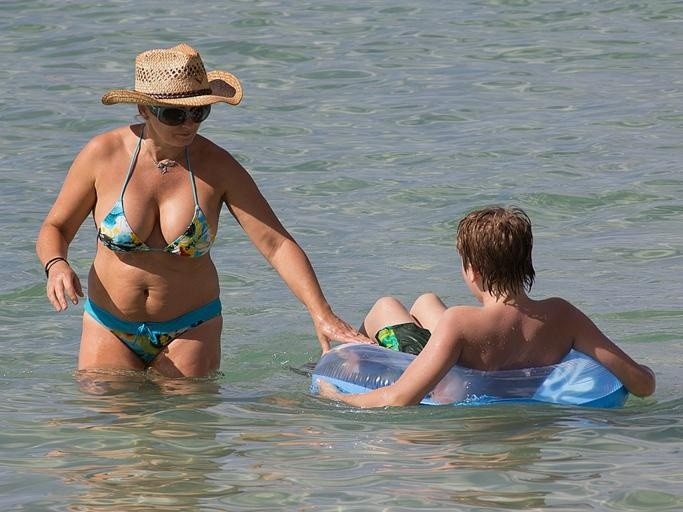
[316,204,655,409]
[35,43,376,378]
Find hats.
[101,43,242,107]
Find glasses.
[147,105,211,126]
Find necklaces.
[147,148,185,175]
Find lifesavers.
[313,342,628,409]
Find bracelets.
[45,257,69,279]
